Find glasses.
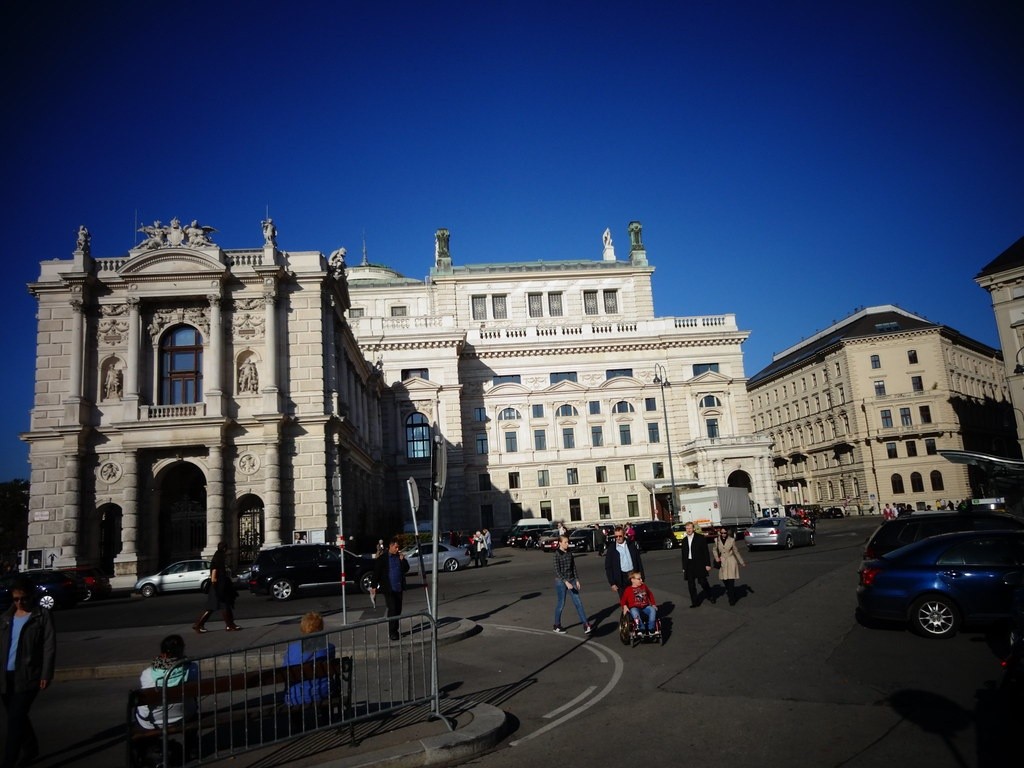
[13,596,25,602]
[635,577,642,580]
[614,535,622,538]
[720,531,727,534]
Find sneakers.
[583,622,597,634]
[553,623,568,634]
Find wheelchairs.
[616,587,663,648]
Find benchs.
[127,657,354,768]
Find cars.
[541,527,578,552]
[399,545,418,555]
[855,530,1024,639]
[743,517,816,552]
[538,529,557,548]
[625,520,674,552]
[59,568,113,608]
[567,528,609,552]
[403,542,471,576]
[670,523,702,546]
[821,508,844,519]
[134,559,213,598]
[0,570,73,612]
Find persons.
[77,225,87,250]
[135,634,201,762]
[449,527,494,568]
[376,538,409,640]
[789,506,817,529]
[0,557,56,768]
[884,495,972,521]
[682,522,716,608]
[146,310,209,337]
[104,463,118,481]
[135,218,216,249]
[192,542,241,634]
[557,521,635,557]
[605,527,645,600]
[282,610,341,707]
[241,455,255,473]
[260,218,277,246]
[621,571,661,637]
[712,526,746,606]
[328,247,347,277]
[105,363,119,398]
[239,357,255,392]
[553,535,597,634]
[603,227,613,246]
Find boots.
[221,608,241,632]
[192,610,211,633]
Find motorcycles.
[506,531,541,550]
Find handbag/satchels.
[713,536,721,569]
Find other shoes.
[690,603,700,608]
[711,600,716,604]
[637,631,642,636]
[389,630,400,640]
[649,631,659,636]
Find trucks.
[679,487,757,538]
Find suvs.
[863,511,1024,561]
[249,544,383,602]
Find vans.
[583,522,615,542]
[502,518,550,543]
